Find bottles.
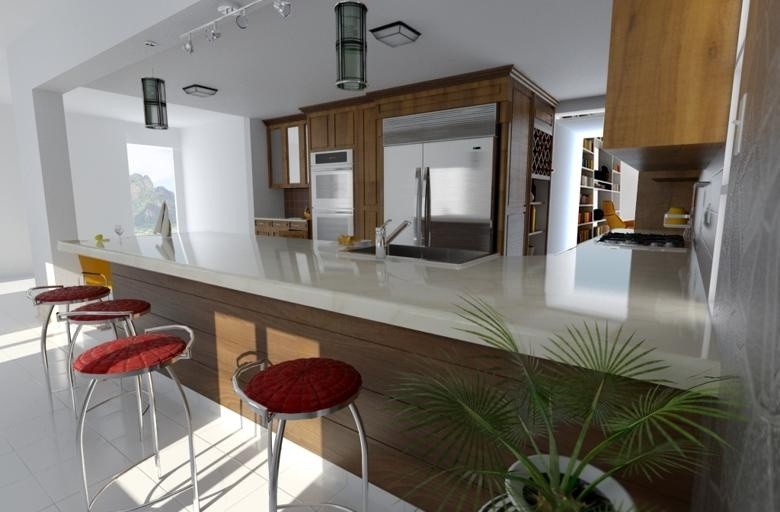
[374,227,386,259]
[579,138,593,242]
[528,179,536,255]
[664,207,687,226]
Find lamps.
[182,82,219,99]
[176,0,293,55]
[142,76,169,132]
[333,0,370,92]
[368,20,423,49]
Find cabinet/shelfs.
[298,95,377,241]
[578,113,622,255]
[255,217,310,239]
[261,113,310,190]
[528,201,546,237]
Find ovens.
[309,168,355,244]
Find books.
[577,140,596,242]
[596,225,609,236]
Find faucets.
[374,219,413,260]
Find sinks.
[344,242,500,270]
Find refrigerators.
[381,103,499,252]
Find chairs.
[602,198,628,229]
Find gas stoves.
[598,232,686,249]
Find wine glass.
[116,225,125,239]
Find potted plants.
[384,287,754,511]
